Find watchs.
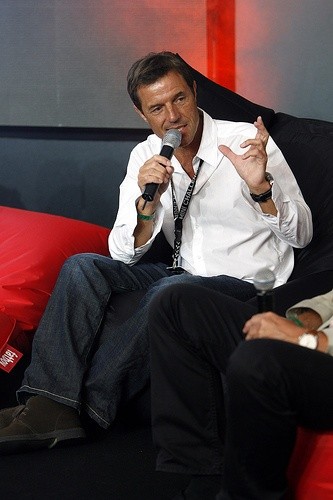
[248,170,276,204]
[297,329,320,350]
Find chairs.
[100,52,333,500]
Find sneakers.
[0,396,85,453]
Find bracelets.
[287,314,304,328]
[139,209,158,223]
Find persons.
[143,276,332,500]
[2,48,314,445]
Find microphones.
[142,129,181,200]
